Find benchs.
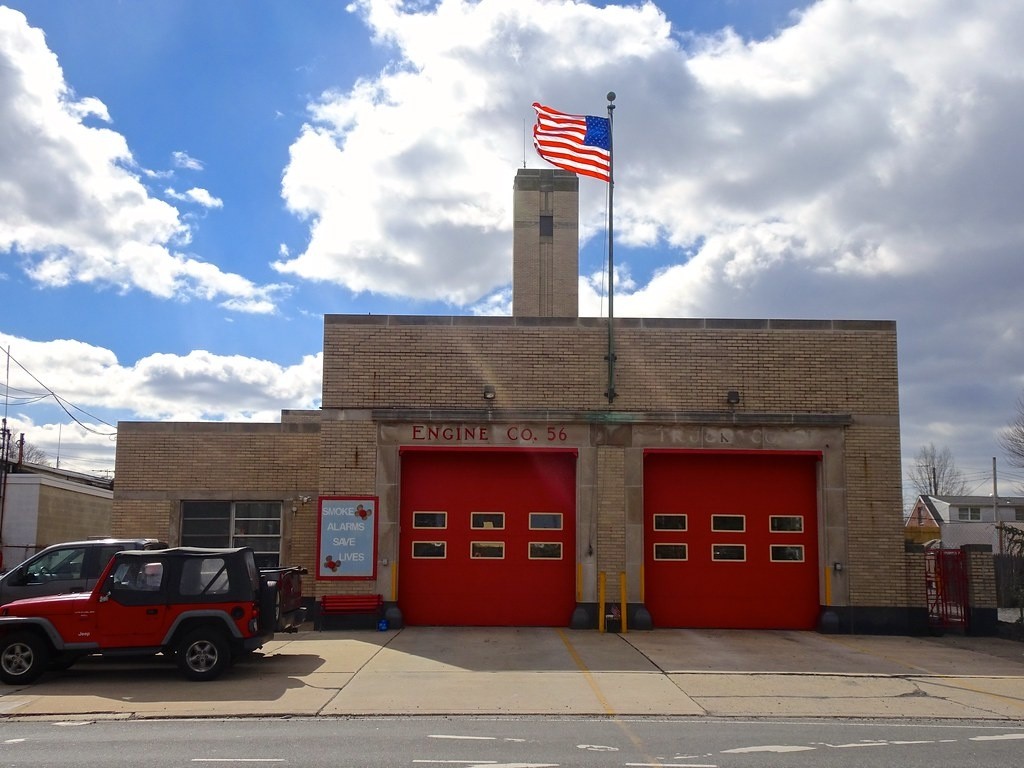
[316,595,384,632]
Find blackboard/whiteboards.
[315,496,380,580]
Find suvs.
[0,547,277,685]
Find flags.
[532,103,611,182]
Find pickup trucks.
[0,539,308,636]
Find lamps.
[484,386,496,399]
[727,392,739,404]
